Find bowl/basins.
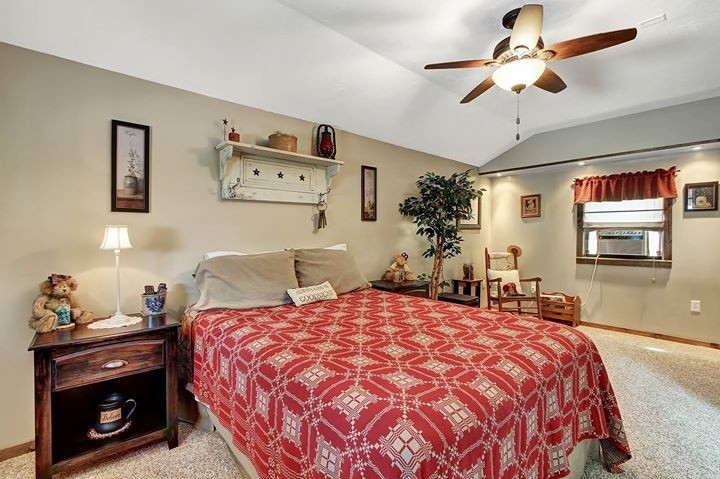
[268,134,298,153]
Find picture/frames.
[110,119,150,213]
[456,197,481,230]
[360,165,377,222]
[685,181,718,212]
[521,194,541,218]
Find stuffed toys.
[383,252,416,282]
[29,274,93,333]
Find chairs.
[485,247,542,319]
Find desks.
[453,279,483,308]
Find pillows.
[286,247,372,295]
[193,248,300,310]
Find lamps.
[87,224,143,329]
[489,58,545,141]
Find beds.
[184,244,599,479]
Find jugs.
[95,392,137,434]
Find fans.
[424,3,637,104]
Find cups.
[141,293,167,315]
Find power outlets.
[690,300,700,313]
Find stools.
[438,292,479,308]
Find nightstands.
[369,278,430,300]
[28,312,183,479]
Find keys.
[318,209,327,229]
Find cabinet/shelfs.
[540,292,580,327]
